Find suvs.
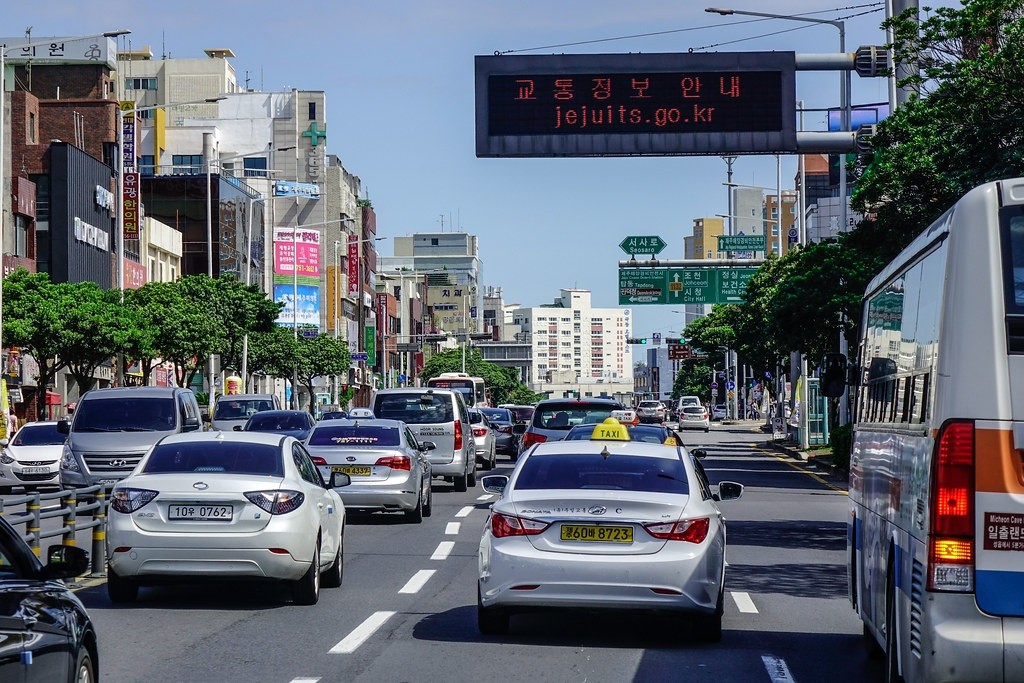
[636,400,667,424]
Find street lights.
[116,97,229,383]
[293,218,388,414]
[243,193,322,393]
[207,145,295,418]
[704,7,848,432]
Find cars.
[678,406,710,433]
[302,408,437,524]
[202,395,283,433]
[712,404,731,421]
[106,429,346,605]
[0,419,74,495]
[234,410,315,443]
[467,388,744,641]
[0,514,99,683]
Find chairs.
[546,418,562,427]
[89,400,128,423]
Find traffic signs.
[667,268,718,304]
[718,267,759,304]
[618,268,668,305]
[717,235,765,252]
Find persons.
[9,408,18,438]
[259,402,268,411]
[750,398,760,420]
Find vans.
[671,396,701,421]
[57,386,205,516]
[368,386,478,492]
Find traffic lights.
[627,338,647,344]
[746,382,753,387]
[664,392,674,395]
[666,338,686,344]
[642,393,652,396]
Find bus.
[819,177,1024,683]
[427,371,488,407]
